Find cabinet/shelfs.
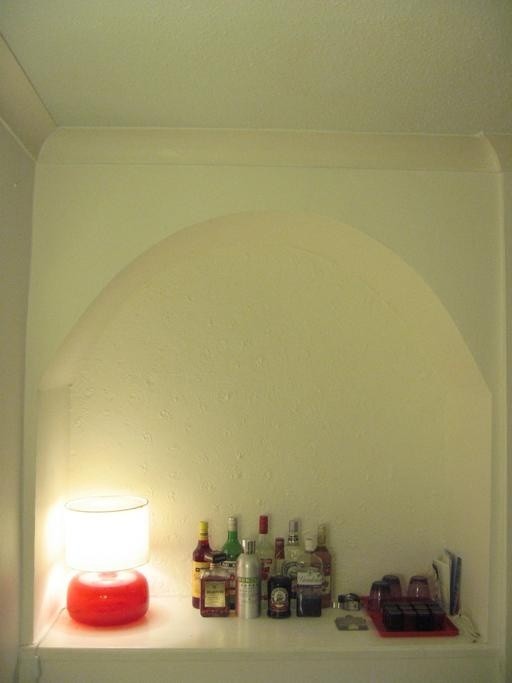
[31,601,493,682]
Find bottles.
[190,514,334,617]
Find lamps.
[57,491,151,627]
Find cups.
[407,575,431,600]
[382,575,401,600]
[367,579,392,611]
